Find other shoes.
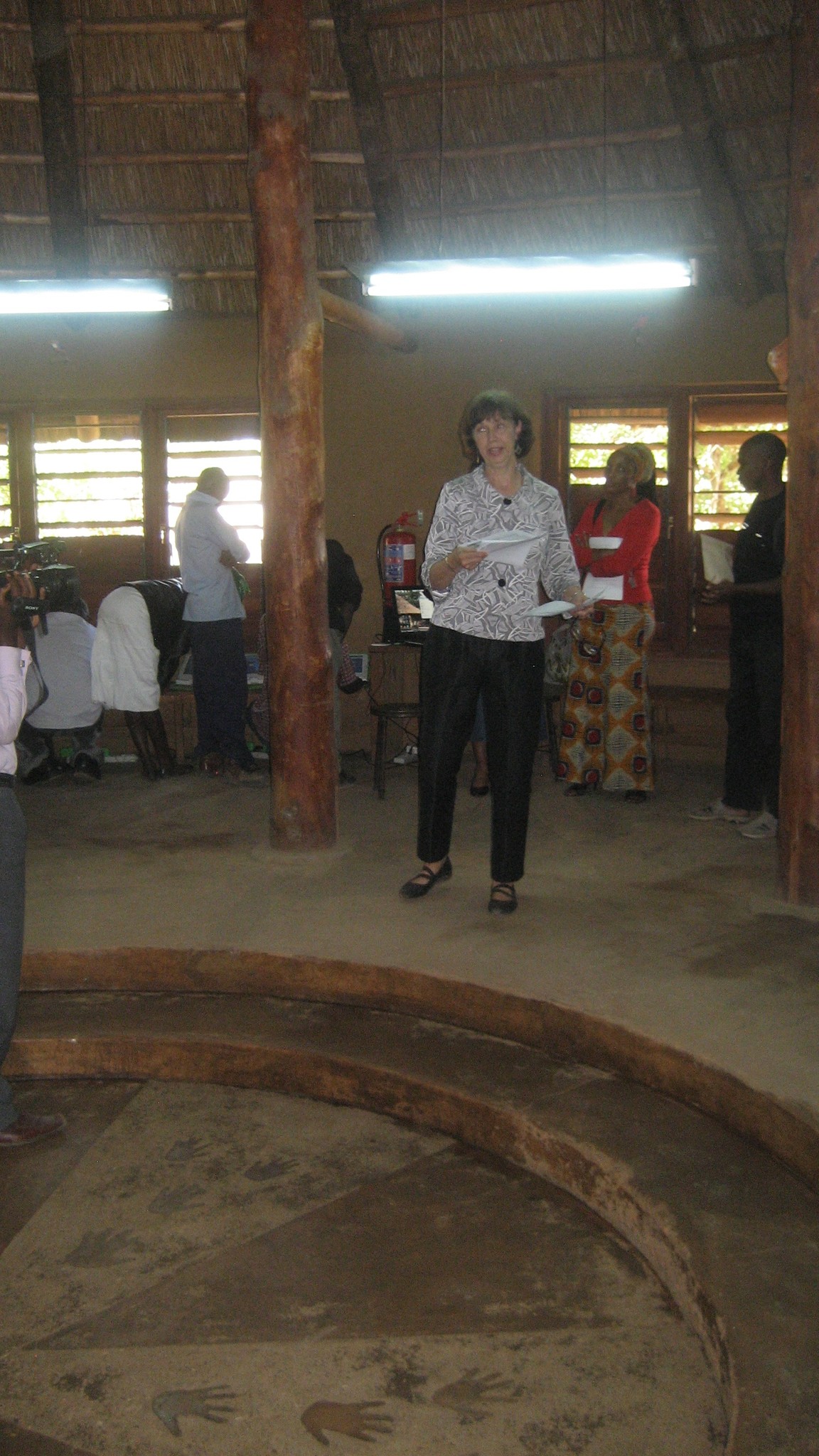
[626,790,646,803]
[143,765,158,780]
[400,857,452,898]
[565,785,596,796]
[73,755,102,783]
[470,774,488,796]
[22,764,63,786]
[488,883,518,916]
[200,757,219,773]
[161,764,194,777]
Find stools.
[369,702,425,798]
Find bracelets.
[445,557,457,574]
[568,590,586,602]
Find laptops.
[391,584,434,647]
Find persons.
[689,433,787,840]
[0,559,70,1152]
[14,594,105,786]
[554,442,662,803]
[399,391,594,915]
[91,575,192,781]
[176,467,370,785]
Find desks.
[370,635,425,767]
[161,683,369,778]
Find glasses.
[603,464,628,477]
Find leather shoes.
[1,1114,65,1146]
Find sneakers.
[740,809,779,838]
[688,797,747,824]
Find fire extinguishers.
[377,511,419,618]
